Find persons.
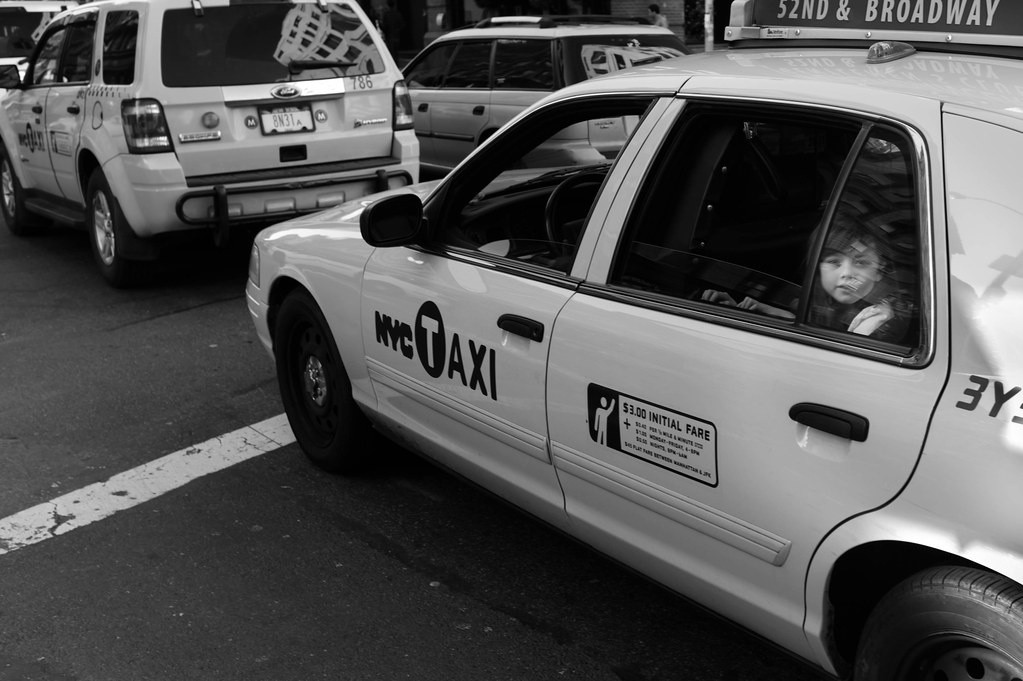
[701,210,907,341]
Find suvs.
[400,15,697,177]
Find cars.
[0,0,421,293]
[252,41,1023,681]
[0,58,67,102]
